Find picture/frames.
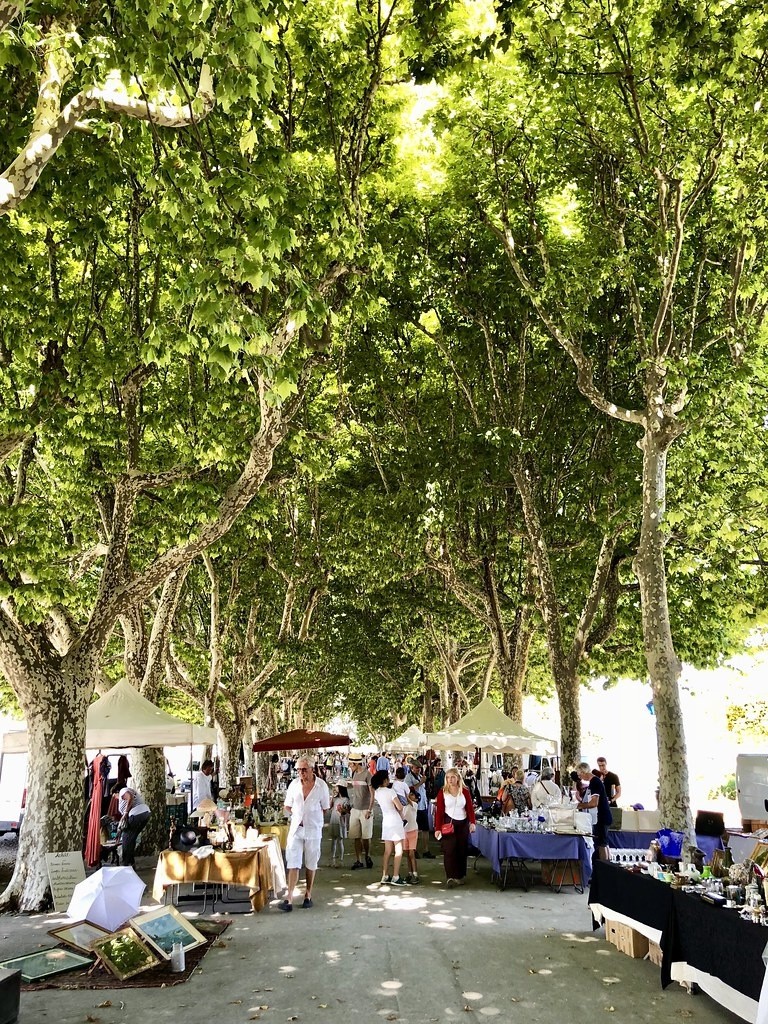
[0,905,208,983]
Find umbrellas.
[66,865,147,933]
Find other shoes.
[325,860,335,867]
[447,878,464,887]
[333,860,344,868]
[351,861,364,870]
[414,850,420,859]
[391,874,407,886]
[404,876,420,884]
[365,856,373,868]
[302,898,313,908]
[278,900,293,912]
[422,851,436,859]
[380,875,392,884]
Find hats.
[542,767,554,776]
[630,803,644,810]
[342,754,365,763]
[331,779,348,787]
[411,760,423,768]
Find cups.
[665,874,673,882]
[658,872,666,879]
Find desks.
[430,803,768,1023]
[151,816,292,915]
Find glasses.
[296,767,310,772]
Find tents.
[382,697,559,775]
[253,727,354,752]
[0,677,218,814]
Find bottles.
[171,942,185,972]
[699,866,715,883]
[683,863,700,881]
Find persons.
[268,750,621,887]
[187,759,213,846]
[112,783,152,869]
[278,756,329,912]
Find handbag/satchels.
[442,823,454,835]
[501,783,515,817]
